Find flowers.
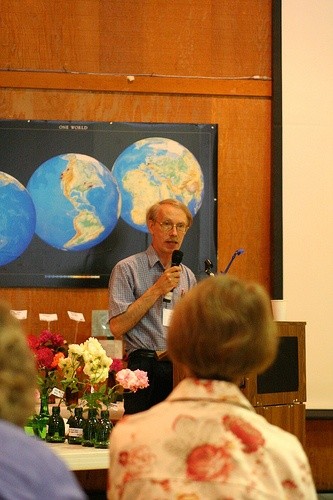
[23,331,68,396]
[57,344,82,407]
[79,337,113,409]
[101,369,149,411]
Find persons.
[105,274,319,500]
[1,300,89,500]
[107,198,198,419]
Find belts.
[128,351,156,359]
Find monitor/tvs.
[0,119,218,289]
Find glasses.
[154,219,189,233]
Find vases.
[46,406,65,443]
[68,407,86,445]
[31,395,50,441]
[83,410,99,447]
[94,411,113,449]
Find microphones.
[163,250,184,303]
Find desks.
[25,434,109,500]
